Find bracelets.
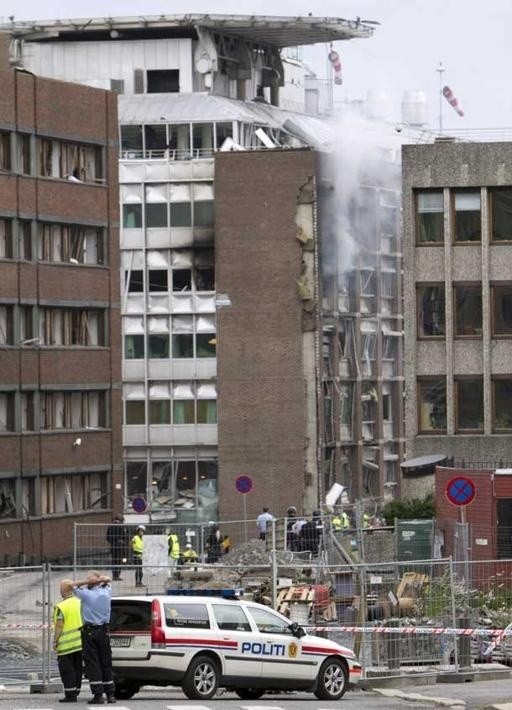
[53,640,58,643]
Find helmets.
[137,525,146,532]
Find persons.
[52,579,84,703]
[68,570,116,704]
[256,506,276,542]
[164,524,230,578]
[284,504,388,560]
[130,524,146,589]
[106,513,126,581]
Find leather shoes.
[88,695,104,704]
[108,696,116,703]
[60,696,77,702]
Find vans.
[83,588,363,700]
